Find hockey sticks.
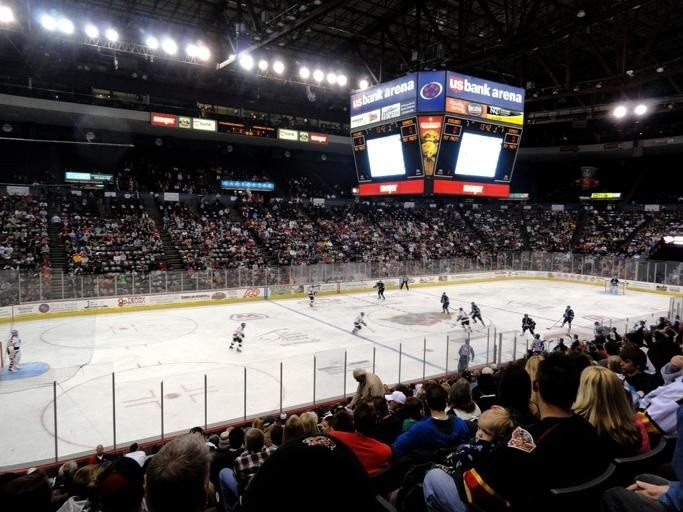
[443,302,458,311]
[546,317,563,330]
[366,325,377,333]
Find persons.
[468,301,486,327]
[455,307,471,333]
[559,306,574,330]
[440,290,449,313]
[350,311,366,334]
[0,313,683,512]
[0,162,683,307]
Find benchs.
[0,183,683,306]
[1,314,683,510]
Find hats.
[242,433,376,512]
[384,390,407,405]
[218,431,230,449]
[94,457,145,512]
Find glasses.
[388,400,393,403]
